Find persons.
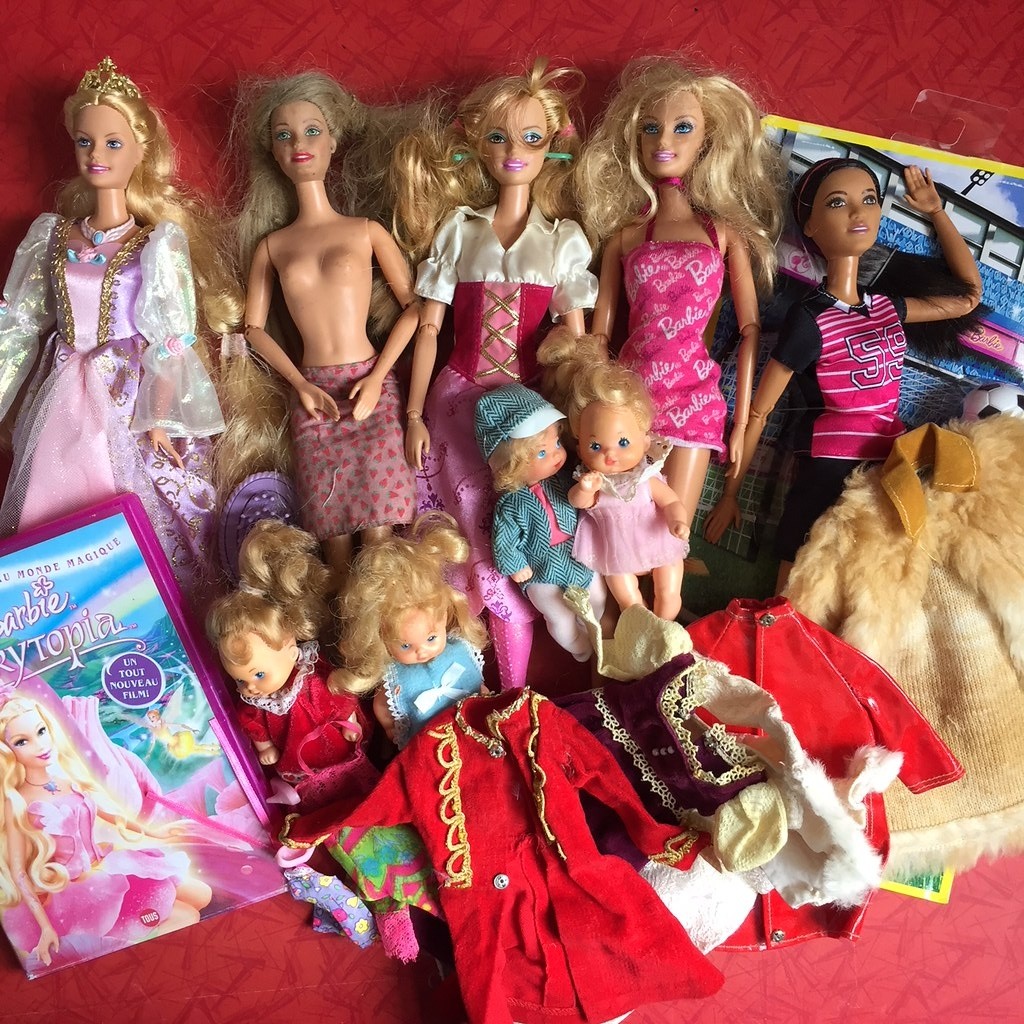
[0,55,985,783]
[0,696,223,966]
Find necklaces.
[80,213,135,246]
[25,777,61,795]
